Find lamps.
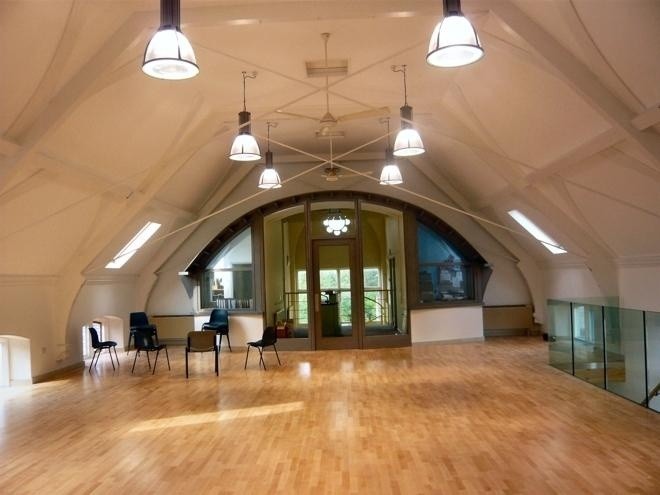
[227,69,265,163]
[142,0,203,83]
[423,0,488,69]
[386,63,427,160]
[323,209,351,238]
[254,120,288,190]
[374,116,404,187]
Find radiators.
[274,309,286,327]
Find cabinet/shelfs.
[319,299,341,337]
[213,289,225,298]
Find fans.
[309,137,374,181]
[273,31,393,136]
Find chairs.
[126,311,152,358]
[202,307,232,353]
[87,326,120,373]
[245,325,282,372]
[183,329,221,380]
[130,328,172,375]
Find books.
[216,296,253,310]
